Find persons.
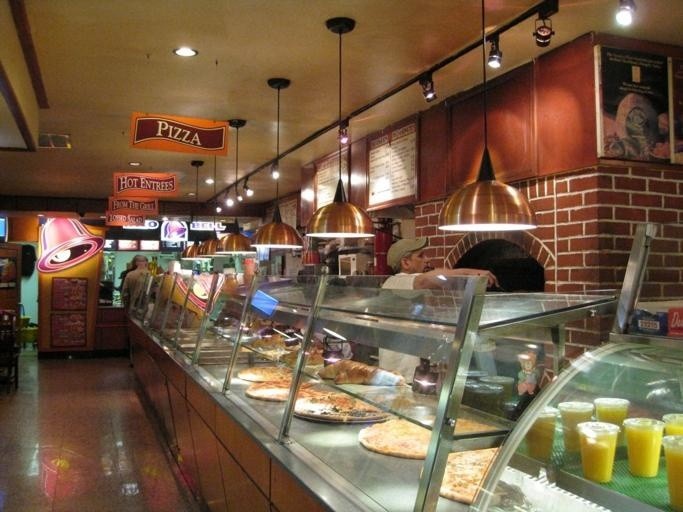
[378,236,500,384]
[120,254,149,310]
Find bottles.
[300,237,319,264]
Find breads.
[252,335,285,349]
[279,350,326,365]
[318,359,406,386]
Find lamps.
[174,0,646,262]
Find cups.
[245,257,256,276]
[526,398,683,510]
[462,371,515,419]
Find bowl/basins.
[20,316,31,328]
[20,327,38,342]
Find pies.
[294,392,389,421]
[420,447,498,504]
[358,415,497,460]
[245,381,336,402]
[238,366,293,382]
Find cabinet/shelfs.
[119,265,683,512]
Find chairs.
[0,308,40,394]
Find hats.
[387,236,429,273]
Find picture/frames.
[47,310,87,349]
[50,276,90,311]
